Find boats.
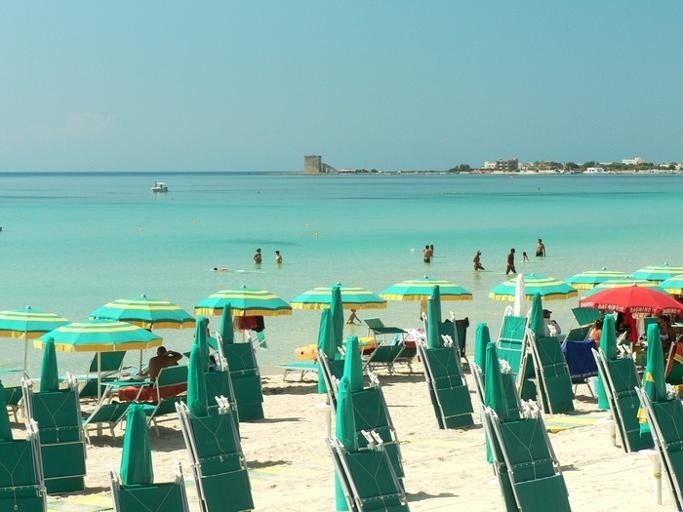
[151,182,168,194]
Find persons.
[422,245,433,264]
[275,250,283,267]
[430,244,434,256]
[506,248,517,274]
[213,268,228,272]
[523,252,529,263]
[473,250,486,271]
[535,239,550,256]
[254,249,262,270]
